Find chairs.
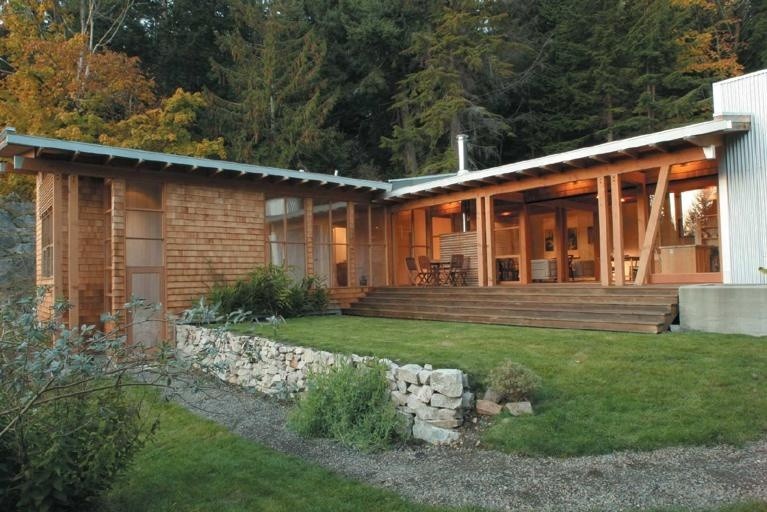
[405,253,469,287]
